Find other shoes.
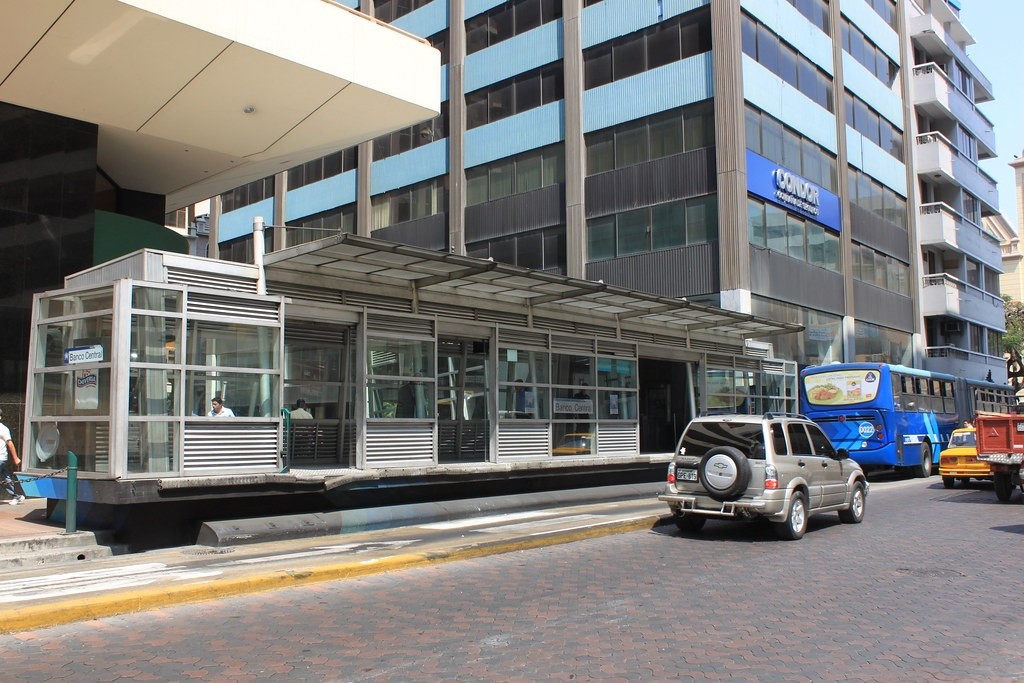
[9,495,25,505]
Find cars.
[939,427,994,487]
[553,435,594,454]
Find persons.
[513,379,534,420]
[573,384,592,449]
[207,396,235,417]
[286,399,314,419]
[0,408,25,507]
[962,418,974,431]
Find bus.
[799,360,1018,478]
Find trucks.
[973,408,1023,500]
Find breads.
[812,387,836,400]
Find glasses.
[963,422,967,425]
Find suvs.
[657,410,872,543]
